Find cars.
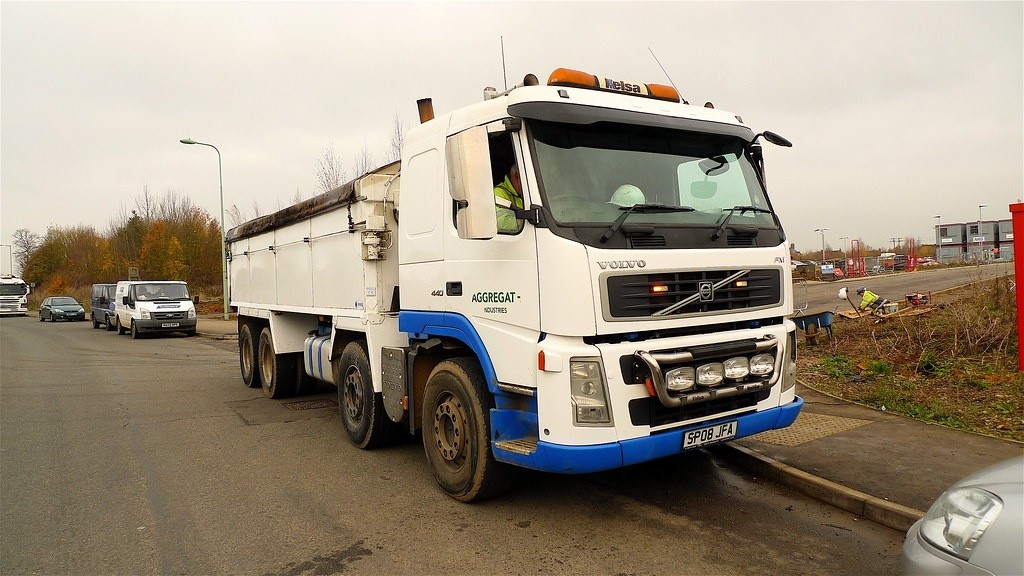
[38,296,86,322]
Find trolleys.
[790,305,840,345]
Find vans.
[115,280,199,339]
[92,285,117,331]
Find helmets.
[856,287,865,295]
[605,184,645,208]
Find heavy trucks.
[227,35,804,504]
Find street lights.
[179,138,228,321]
[815,228,829,265]
[980,205,988,262]
[839,237,848,260]
[933,215,942,264]
[0,245,13,275]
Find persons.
[494,163,523,231]
[856,286,890,314]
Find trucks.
[0,276,30,316]
